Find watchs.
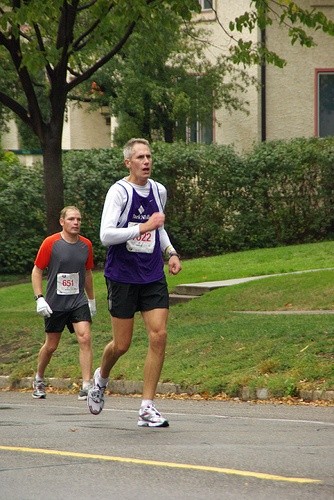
[169,253,181,260]
[34,294,44,301]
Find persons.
[88,138,180,427]
[31,205,94,400]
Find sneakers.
[137,404,169,427]
[88,367,109,415]
[77,385,89,401]
[32,376,46,398]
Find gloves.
[36,297,53,317]
[88,299,97,318]
